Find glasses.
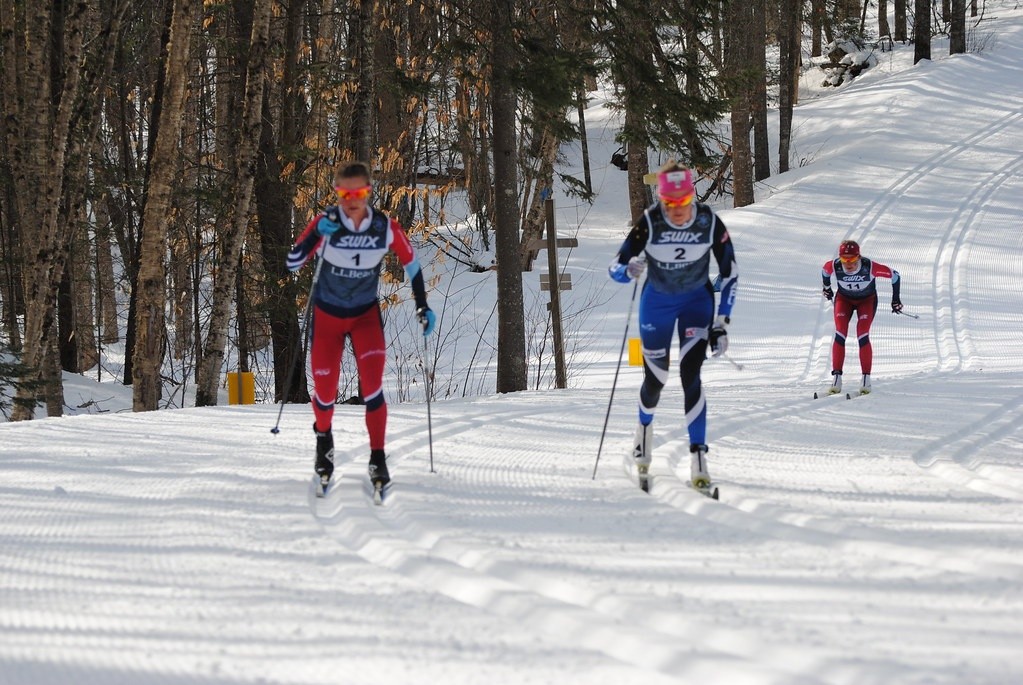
[840,256,859,263]
[659,191,694,207]
[334,185,371,200]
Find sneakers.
[828,374,843,394]
[369,449,390,490]
[860,374,871,394]
[315,435,335,478]
[634,422,655,467]
[690,444,710,488]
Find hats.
[840,241,860,257]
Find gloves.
[626,257,645,278]
[891,300,902,314]
[416,303,436,336]
[316,218,340,239]
[822,288,834,300]
[709,320,728,357]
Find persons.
[608,163,739,486]
[822,241,903,394]
[286,162,432,505]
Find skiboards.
[634,478,719,500]
[309,467,390,508]
[813,386,873,400]
